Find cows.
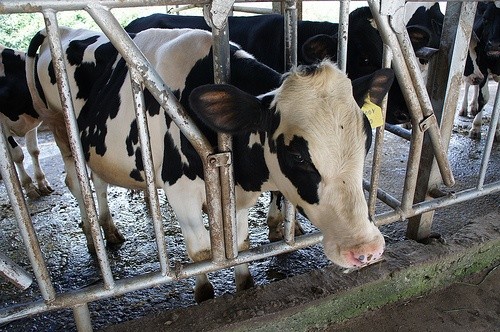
[0,0,500,305]
